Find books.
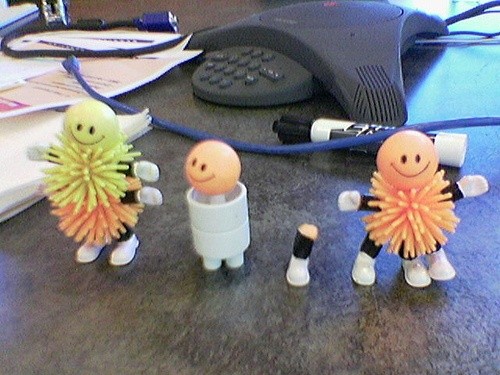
[0,30,207,122]
[0,104,156,226]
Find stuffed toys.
[336,127,488,293]
[24,98,164,267]
